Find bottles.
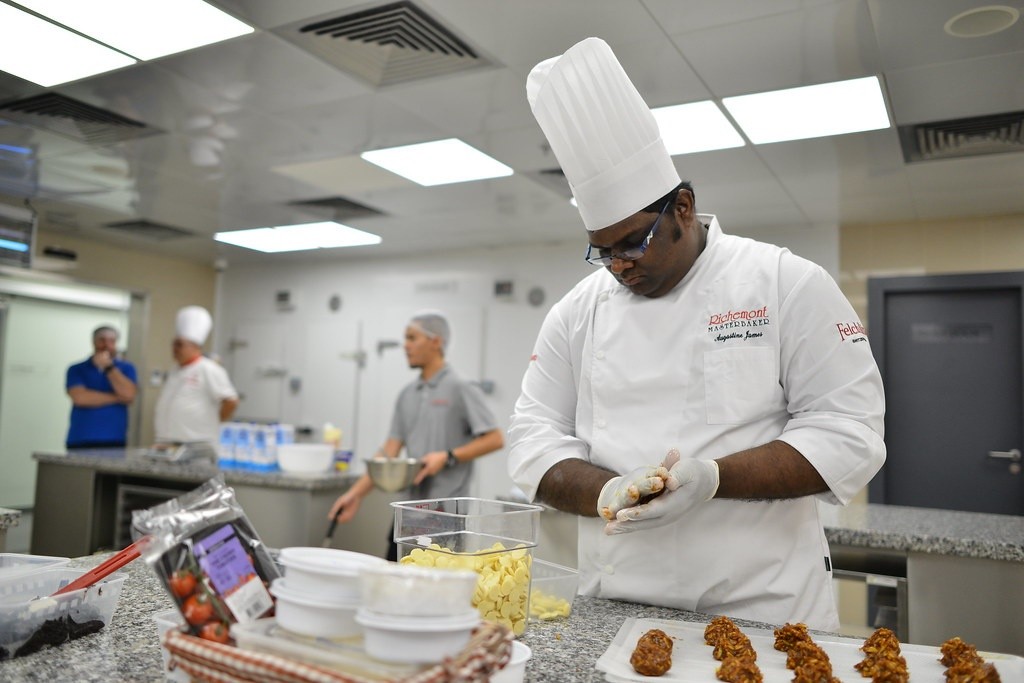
[217,420,292,474]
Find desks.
[0,552,1024,683]
[28,450,394,557]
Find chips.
[532,586,571,620]
[400,542,531,636]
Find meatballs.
[631,616,1002,683]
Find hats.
[525,37,686,233]
[171,302,213,345]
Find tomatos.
[201,622,227,643]
[184,594,212,621]
[170,571,195,596]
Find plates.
[596,616,1024,683]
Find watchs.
[445,449,458,470]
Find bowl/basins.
[364,565,480,616]
[275,547,385,604]
[364,456,422,494]
[353,611,483,664]
[276,443,335,474]
[269,577,359,639]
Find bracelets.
[103,363,116,377]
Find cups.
[490,640,533,683]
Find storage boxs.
[390,496,544,640]
[169,634,531,683]
[527,560,580,622]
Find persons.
[328,313,506,562]
[508,37,888,632]
[64,327,139,449]
[153,307,239,463]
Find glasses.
[585,203,673,266]
